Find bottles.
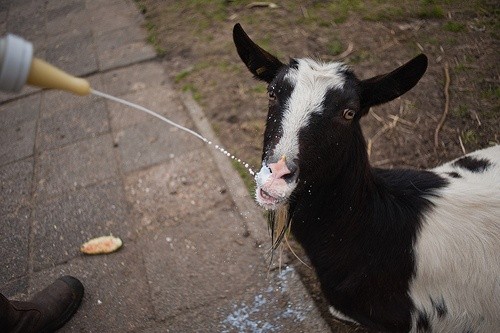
[0,34,89,95]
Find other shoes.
[0,275,86,331]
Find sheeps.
[232,23,500,333]
